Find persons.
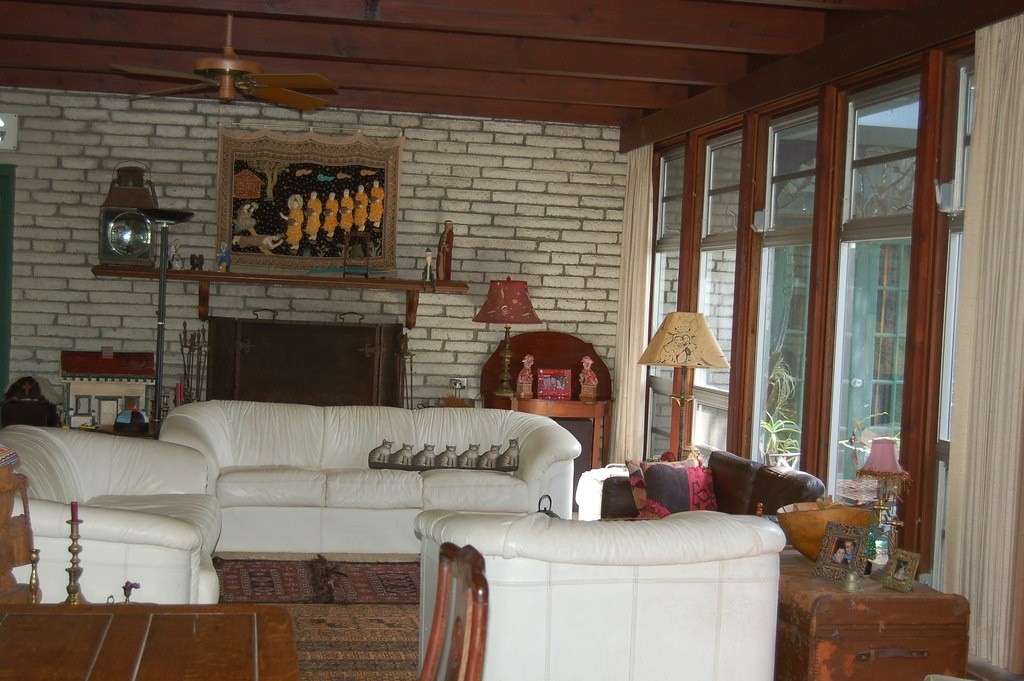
[894,561,907,581]
[437,220,454,280]
[217,242,231,273]
[834,539,855,564]
[168,239,184,269]
[544,375,565,391]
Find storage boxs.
[773,547,971,681]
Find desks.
[1,605,303,681]
[490,393,612,469]
[2,399,57,425]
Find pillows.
[624,459,717,518]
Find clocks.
[98,162,156,267]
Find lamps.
[855,436,904,580]
[139,206,196,421]
[472,276,542,397]
[636,312,733,462]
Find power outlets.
[451,378,468,389]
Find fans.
[108,13,339,111]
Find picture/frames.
[536,368,573,400]
[882,550,920,594]
[812,522,869,580]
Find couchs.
[601,448,826,523]
[159,398,582,554]
[0,424,222,605]
[414,507,787,681]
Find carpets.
[212,557,421,606]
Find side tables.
[97,423,161,439]
